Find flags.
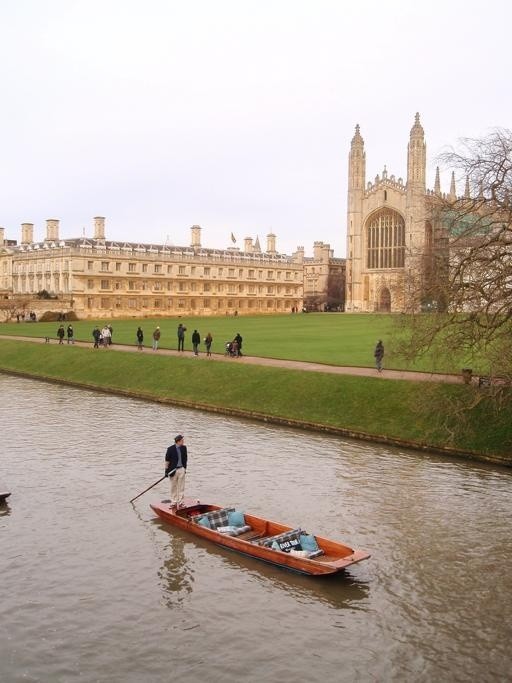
[231,233,236,243]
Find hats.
[175,435,184,442]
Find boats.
[0,491,11,500]
[149,500,371,578]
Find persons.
[91,323,113,348]
[191,329,200,356]
[292,304,344,314]
[165,435,188,509]
[17,310,66,323]
[177,323,187,353]
[234,333,243,358]
[67,325,75,345]
[152,326,161,352]
[205,332,212,356]
[232,339,239,358]
[137,326,144,351]
[373,339,385,373]
[57,325,64,344]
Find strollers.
[224,342,236,358]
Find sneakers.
[179,503,186,509]
[169,503,177,509]
